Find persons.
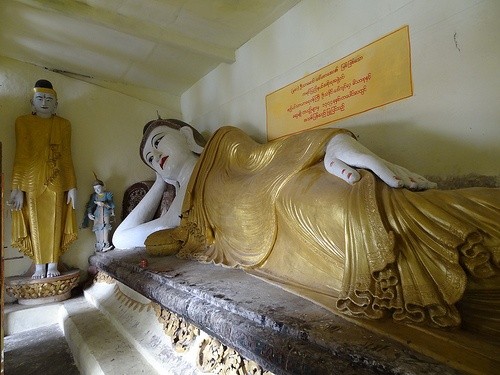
[111,118,499,328]
[81,169,116,253]
[8,78,79,279]
